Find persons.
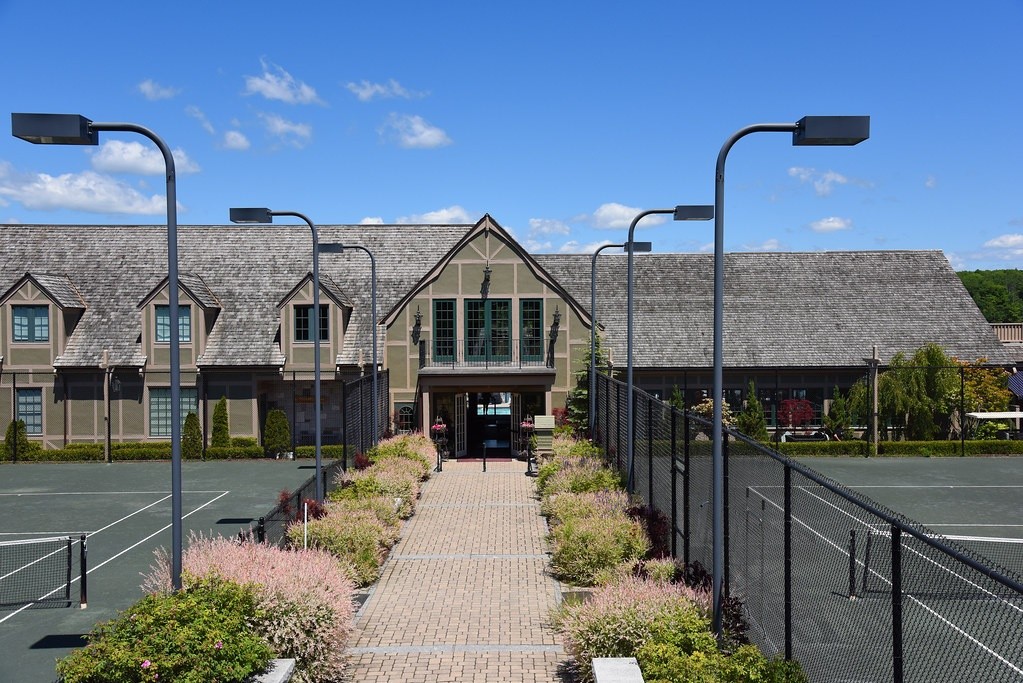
[482,392,493,415]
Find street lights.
[315,241,380,456]
[585,242,652,437]
[704,115,871,619]
[8,109,187,595]
[223,205,328,513]
[624,206,715,493]
